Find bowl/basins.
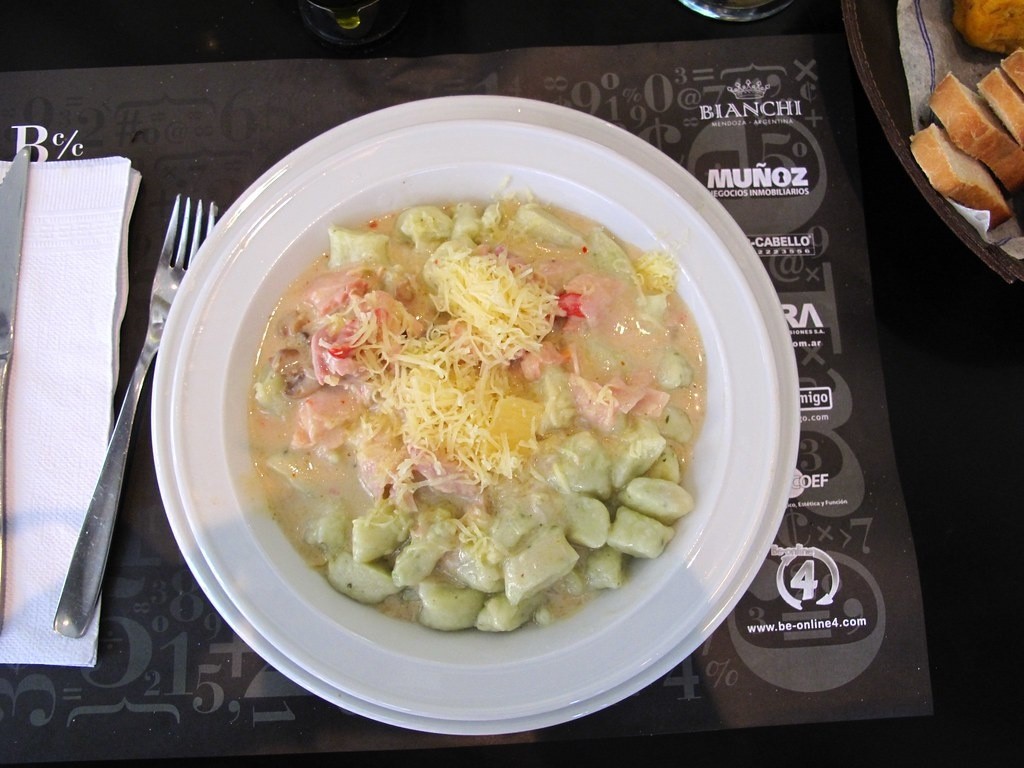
[150,93,801,736]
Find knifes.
[0,145,33,635]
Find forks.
[53,194,217,637]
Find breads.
[908,0,1024,232]
